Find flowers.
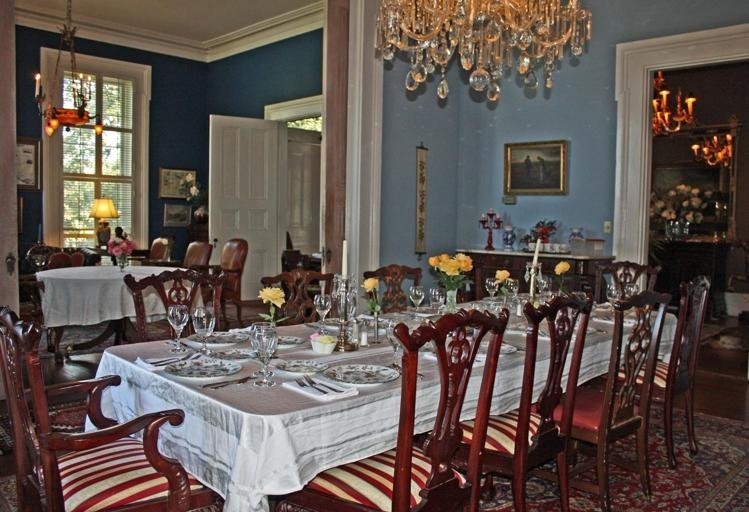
[648,184,712,224]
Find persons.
[525,156,531,176]
[537,156,545,180]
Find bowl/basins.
[309,334,339,356]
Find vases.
[666,219,688,239]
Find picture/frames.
[16,136,42,193]
[503,140,568,195]
[158,167,196,202]
[164,204,191,228]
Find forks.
[295,374,342,398]
[150,349,196,366]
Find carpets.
[0,400,749,512]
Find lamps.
[374,0,592,101]
[33,0,102,137]
[692,134,734,172]
[652,71,696,135]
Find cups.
[527,242,571,251]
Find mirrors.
[648,124,742,244]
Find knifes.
[390,362,429,382]
[203,374,256,391]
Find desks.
[655,233,731,320]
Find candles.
[482,208,500,221]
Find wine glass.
[312,276,639,346]
[165,304,215,357]
[249,321,278,387]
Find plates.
[164,328,330,380]
[476,342,518,354]
[323,364,401,384]
